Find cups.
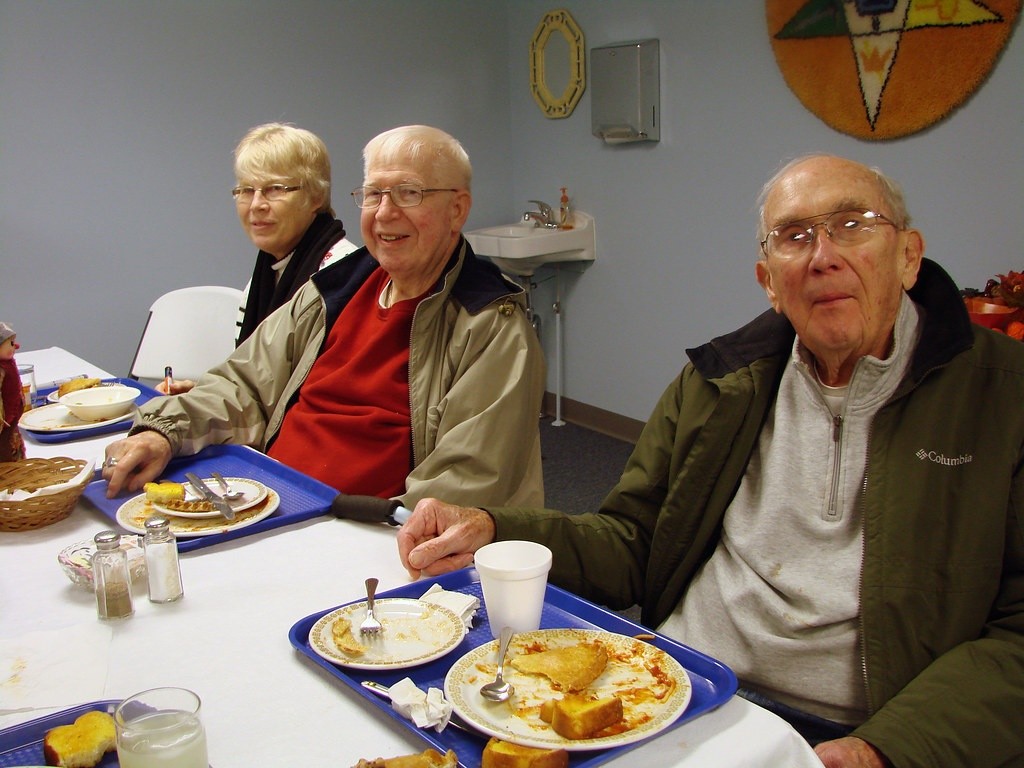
[114,686,209,768]
[13,363,39,403]
[473,539,553,638]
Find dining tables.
[0,345,826,768]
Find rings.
[107,457,119,467]
[102,462,106,470]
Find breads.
[144,481,186,503]
[539,694,624,740]
[57,377,101,398]
[481,737,570,768]
[43,711,127,768]
[166,499,212,512]
[510,643,610,692]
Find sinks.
[461,208,595,277]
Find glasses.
[761,207,904,259]
[233,183,304,202]
[351,184,458,208]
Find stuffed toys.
[0,323,26,464]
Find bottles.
[89,530,135,619]
[142,517,183,604]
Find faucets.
[523,198,553,227]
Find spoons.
[209,470,242,500]
[360,577,383,637]
[481,627,513,703]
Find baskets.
[0,457,92,532]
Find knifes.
[184,472,236,521]
[363,679,491,742]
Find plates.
[309,597,466,670]
[16,402,137,432]
[116,484,281,537]
[444,628,691,751]
[48,382,128,403]
[151,479,267,520]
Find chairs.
[131,285,243,384]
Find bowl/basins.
[57,539,146,593]
[57,384,141,420]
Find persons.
[101,122,546,513]
[397,154,1023,767]
[154,123,359,397]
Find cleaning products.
[559,187,573,229]
